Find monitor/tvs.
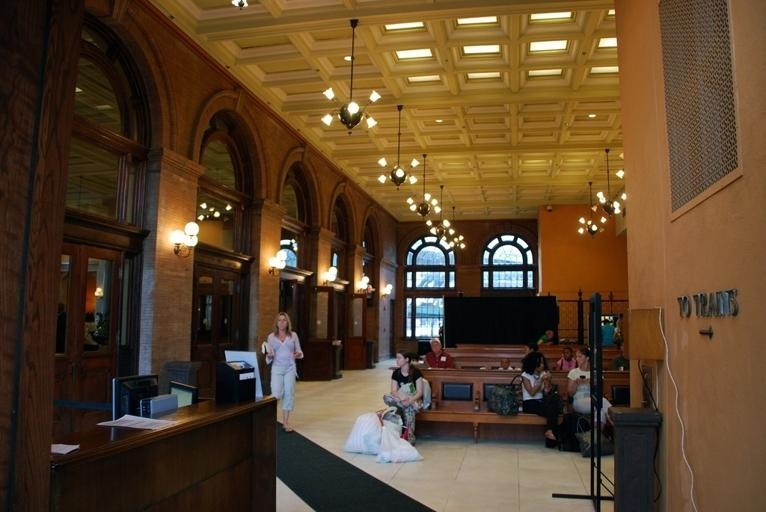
[169,380,198,408]
[111,374,160,422]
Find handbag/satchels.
[487,385,518,416]
[575,415,613,458]
[503,375,525,413]
[380,406,408,436]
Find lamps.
[198,201,233,222]
[170,222,199,258]
[357,276,369,294]
[321,266,337,286]
[591,149,626,218]
[382,284,392,296]
[406,154,441,221]
[377,105,420,190]
[321,19,381,135]
[577,182,607,240]
[267,250,288,278]
[446,207,466,250]
[426,185,455,238]
[625,309,665,408]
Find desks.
[48,395,279,512]
[607,406,661,512]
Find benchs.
[444,343,618,369]
[415,371,630,443]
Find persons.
[524,343,549,370]
[566,347,614,432]
[383,350,424,446]
[613,338,630,370]
[556,347,577,372]
[538,330,553,345]
[617,312,624,337]
[601,319,616,345]
[425,339,454,369]
[411,352,427,368]
[266,313,304,432]
[615,328,621,337]
[521,352,562,440]
[498,358,513,370]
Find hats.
[429,337,440,344]
